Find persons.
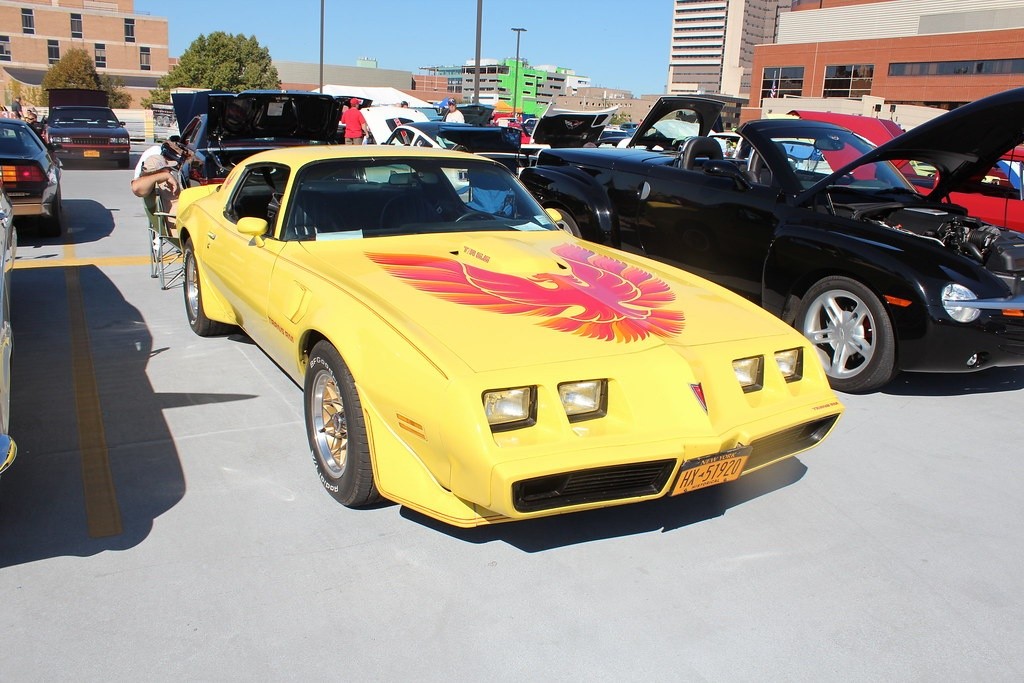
[11,96,24,119]
[445,98,465,123]
[341,98,369,145]
[131,152,181,230]
[27,110,37,122]
[400,101,408,108]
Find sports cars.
[178,144,846,529]
[356,105,538,186]
[519,84,1024,394]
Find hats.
[448,98,456,105]
[141,153,178,175]
[350,98,363,105]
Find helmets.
[401,101,408,105]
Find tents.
[491,99,523,116]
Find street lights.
[512,28,527,118]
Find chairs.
[269,190,346,240]
[673,135,723,173]
[381,194,443,230]
[145,181,185,290]
[746,142,788,187]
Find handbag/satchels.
[361,136,368,145]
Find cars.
[0,119,62,236]
[493,103,640,149]
[0,191,19,480]
[170,90,340,190]
[46,106,130,168]
